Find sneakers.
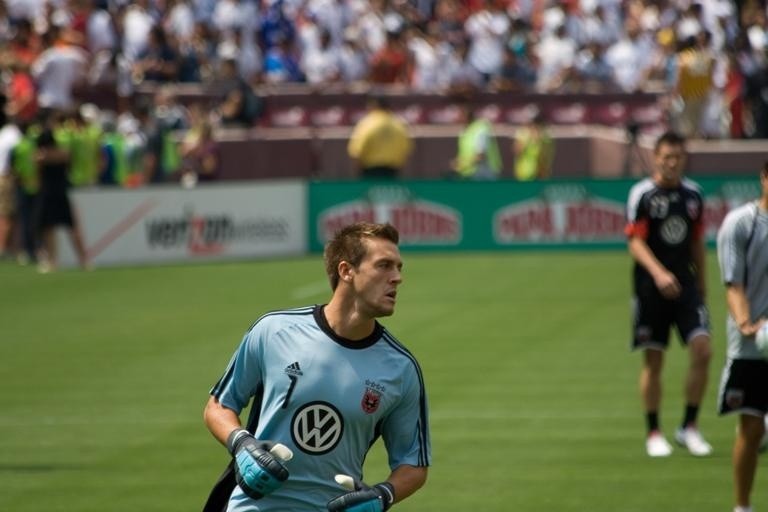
[647,430,673,457]
[674,423,713,457]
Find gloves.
[227,429,293,500]
[327,474,395,512]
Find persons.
[712,159,768,512]
[200,217,435,511]
[347,94,412,182]
[454,103,557,180]
[1,103,223,187]
[1,0,767,140]
[1,129,93,270]
[621,129,716,460]
